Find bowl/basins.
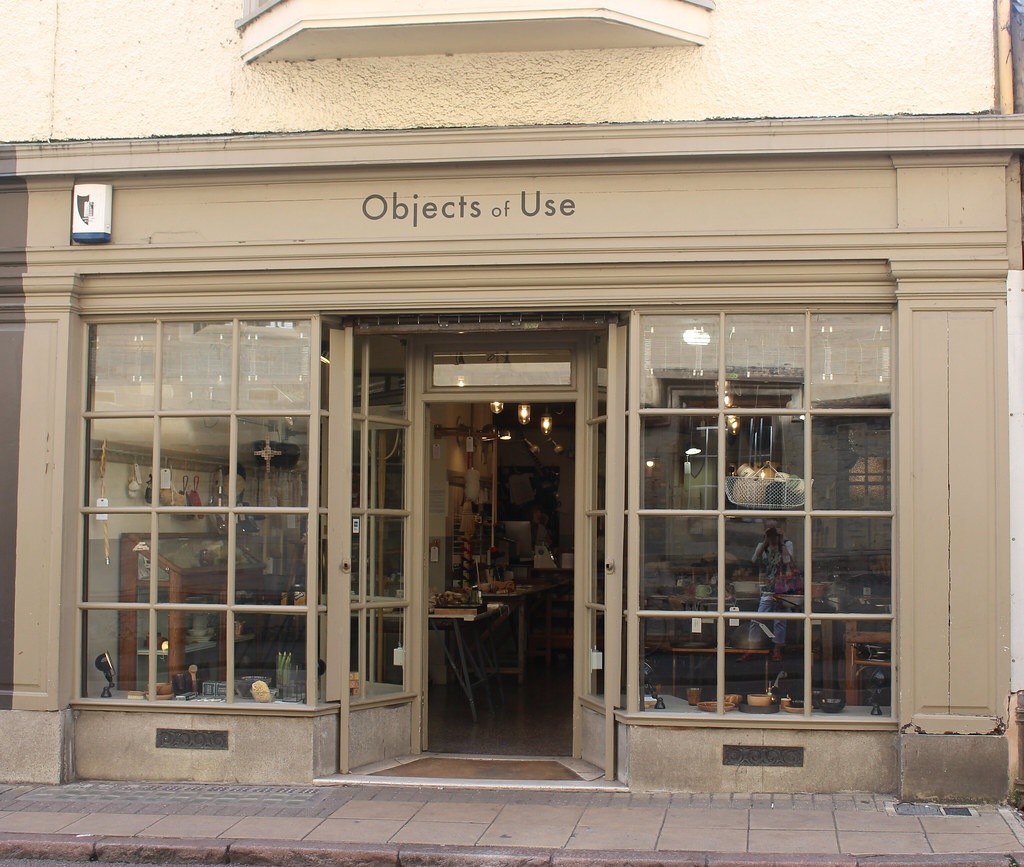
[724,693,742,706]
[147,683,172,696]
[817,699,846,713]
[790,700,806,707]
[233,675,272,700]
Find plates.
[696,702,735,712]
[785,705,814,714]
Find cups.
[686,687,701,707]
[172,672,193,697]
[282,668,307,703]
[780,698,791,709]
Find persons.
[738,520,794,663]
[527,503,554,548]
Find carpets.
[367,759,588,779]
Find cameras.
[766,528,778,538]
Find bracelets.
[779,543,786,547]
[763,541,768,545]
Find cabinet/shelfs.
[119,532,269,691]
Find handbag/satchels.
[775,552,803,594]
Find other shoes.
[736,653,755,664]
[771,648,784,662]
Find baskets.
[725,461,814,509]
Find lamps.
[523,437,564,455]
[683,442,701,474]
[457,424,511,443]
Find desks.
[272,584,893,723]
